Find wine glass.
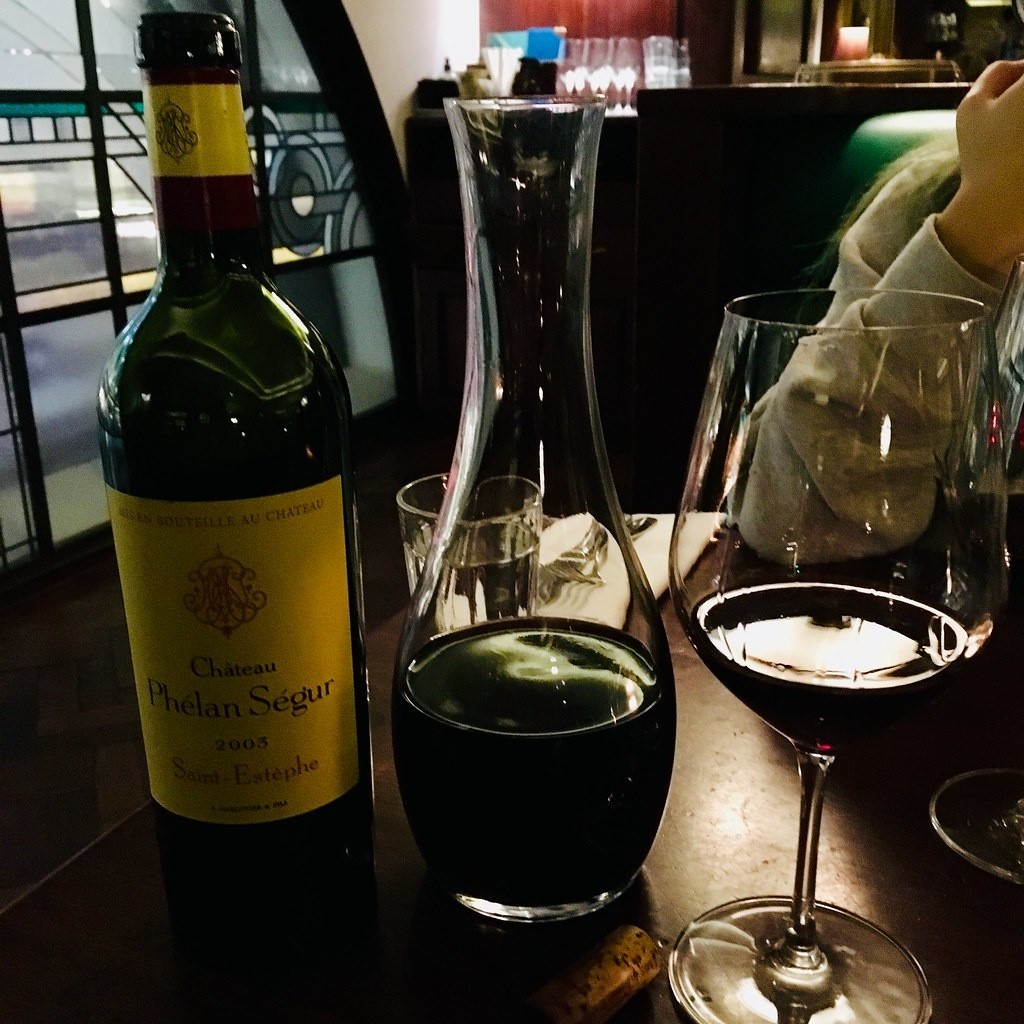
[557,37,640,116]
[666,287,1012,1024]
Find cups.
[642,35,691,88]
[397,473,542,640]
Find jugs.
[390,95,681,925]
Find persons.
[722,58,1023,568]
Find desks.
[0,507,1024,1023]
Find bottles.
[95,11,380,949]
